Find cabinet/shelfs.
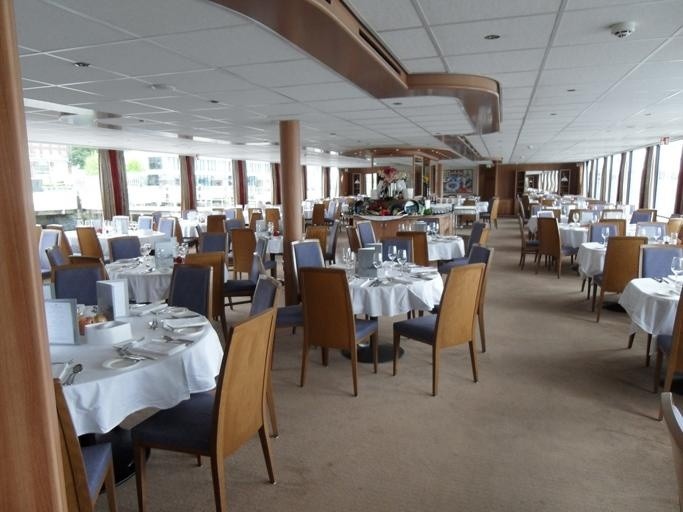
[558,169,570,196]
[514,168,525,218]
[350,172,362,196]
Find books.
[366,242,382,264]
[42,282,56,299]
[44,297,80,346]
[354,247,377,278]
[94,278,130,323]
[255,220,264,233]
[151,236,176,263]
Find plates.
[101,357,137,370]
[162,305,186,316]
[172,327,204,335]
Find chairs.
[297,266,380,397]
[427,243,494,354]
[130,305,279,511]
[32,192,500,306]
[657,390,682,448]
[51,377,120,511]
[252,252,302,368]
[164,262,216,325]
[390,262,486,399]
[244,273,284,440]
[513,187,682,422]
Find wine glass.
[341,244,408,282]
[397,222,440,241]
[669,255,683,285]
[538,194,678,249]
[138,240,190,267]
[76,217,114,234]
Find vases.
[384,182,396,198]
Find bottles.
[75,306,108,337]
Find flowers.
[376,168,408,192]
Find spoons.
[161,335,192,345]
[63,363,82,386]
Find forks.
[112,336,157,362]
